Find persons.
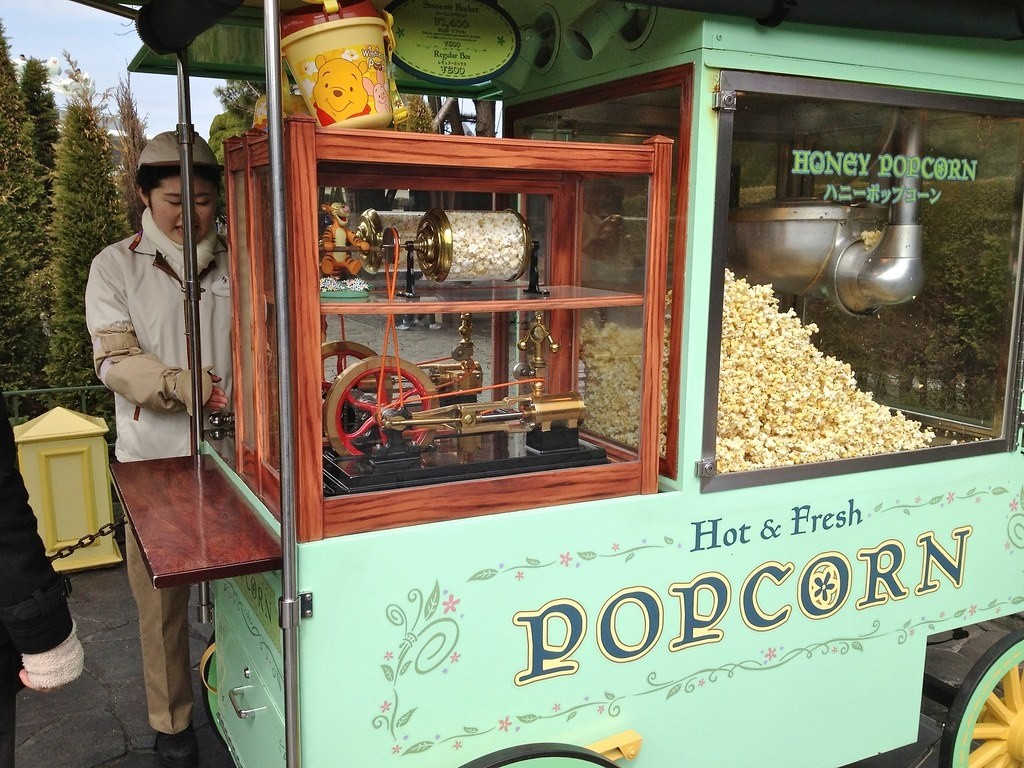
[85,130,233,768]
[0,393,82,768]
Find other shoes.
[154,723,199,768]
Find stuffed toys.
[321,200,370,273]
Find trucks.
[70,1,1024,768]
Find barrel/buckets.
[279,1,396,128]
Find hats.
[137,130,224,172]
